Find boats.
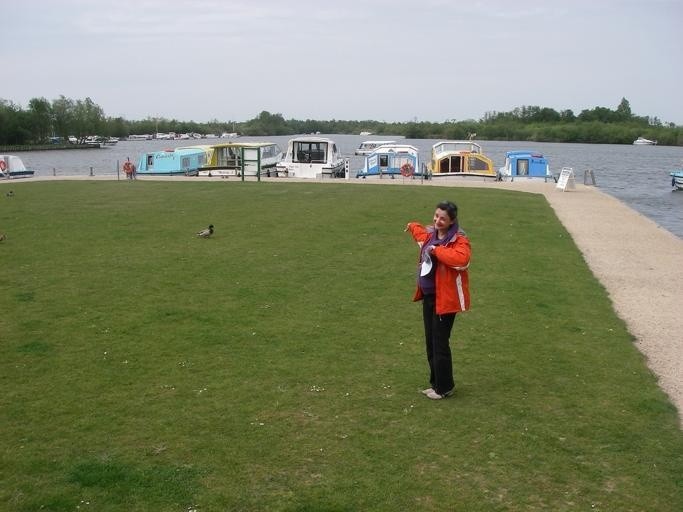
[631,136,657,146]
[425,142,497,182]
[354,141,395,156]
[48,132,241,144]
[497,150,553,183]
[276,136,346,179]
[133,146,213,179]
[197,142,284,179]
[667,171,683,191]
[0,155,35,181]
[356,145,426,181]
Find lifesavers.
[0,160,7,171]
[124,162,133,173]
[400,164,414,176]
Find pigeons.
[196,224,216,239]
[0,232,8,242]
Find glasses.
[442,199,457,216]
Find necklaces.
[437,232,448,235]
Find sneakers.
[422,387,457,399]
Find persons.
[123,157,133,178]
[401,200,472,400]
